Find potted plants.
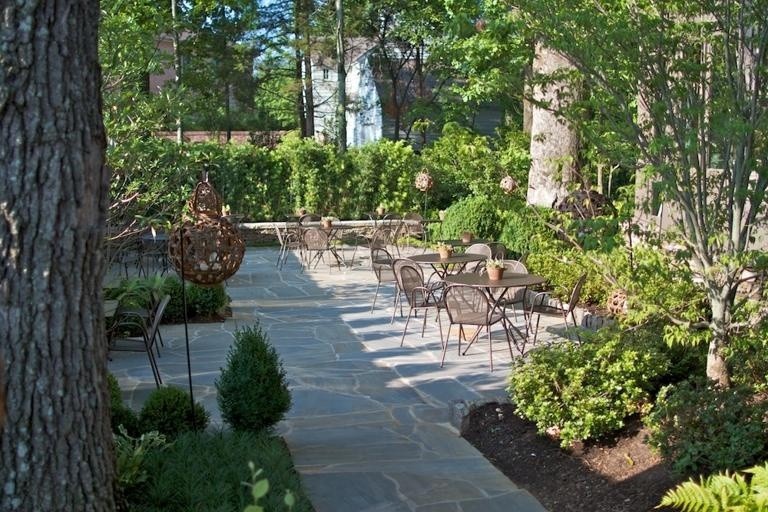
[484,256,507,282]
[375,203,386,214]
[459,227,476,246]
[292,206,306,214]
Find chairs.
[439,283,517,373]
[368,248,406,320]
[519,270,589,355]
[399,266,468,353]
[272,213,432,277]
[391,257,443,325]
[105,286,172,390]
[465,240,508,277]
[475,259,531,344]
[109,235,174,281]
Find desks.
[435,237,490,276]
[405,251,488,322]
[443,269,551,372]
